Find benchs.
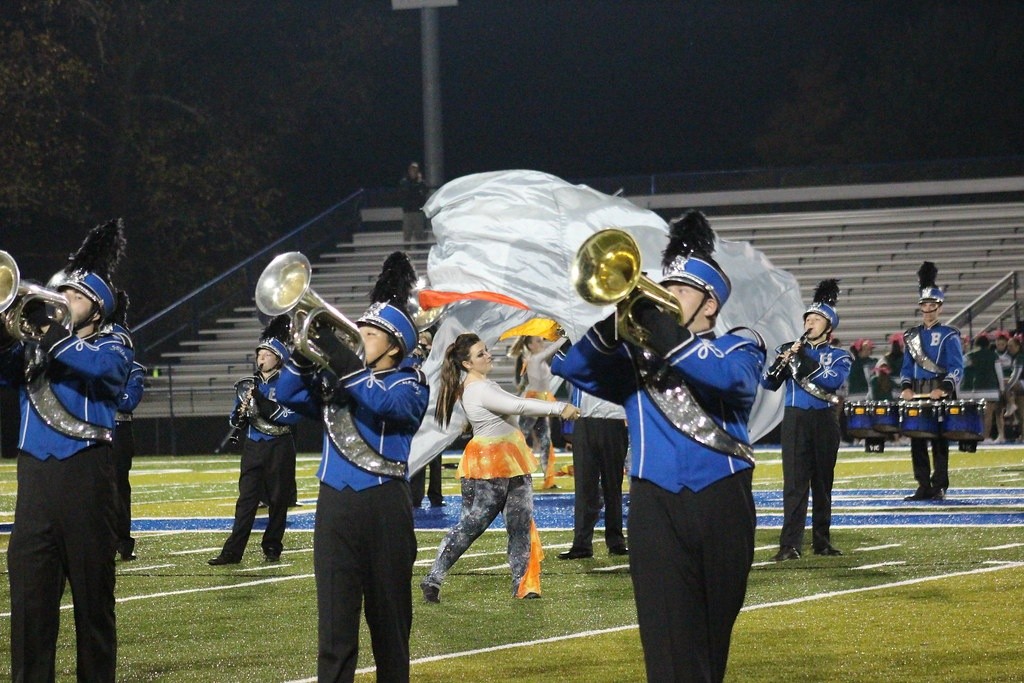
[131,176,1024,413]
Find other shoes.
[209,552,240,564]
[420,583,441,603]
[431,501,446,507]
[932,487,946,504]
[526,592,539,598]
[984,438,995,445]
[1004,403,1017,416]
[904,486,932,501]
[994,437,1006,444]
[288,502,302,507]
[257,501,267,508]
[267,547,279,561]
[126,555,136,560]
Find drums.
[869,401,902,433]
[842,400,886,438]
[941,400,985,442]
[899,400,940,439]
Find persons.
[306,300,431,682]
[0,269,137,683]
[400,162,430,259]
[203,253,1023,599]
[552,257,768,683]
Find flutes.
[228,362,265,446]
[767,327,812,383]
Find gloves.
[251,387,266,407]
[312,317,354,362]
[4,294,52,334]
[244,414,258,423]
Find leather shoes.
[769,545,800,561]
[558,550,593,559]
[814,547,842,556]
[609,546,630,554]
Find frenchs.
[405,273,448,360]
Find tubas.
[571,227,685,356]
[254,249,368,378]
[0,249,74,347]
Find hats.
[657,209,731,316]
[918,261,947,306]
[57,217,131,325]
[804,279,840,329]
[355,251,419,355]
[256,313,292,364]
